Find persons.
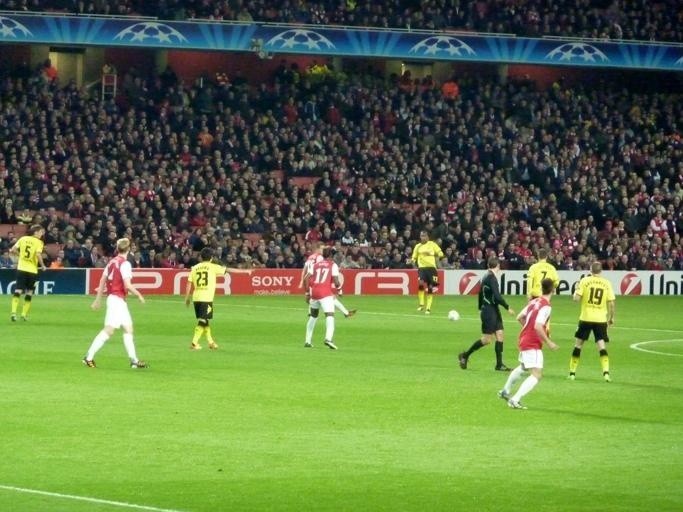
[496,277,560,410]
[21,59,44,242]
[568,264,615,383]
[8,224,45,322]
[305,247,342,349]
[0,60,21,268]
[457,256,514,372]
[314,57,331,249]
[525,248,560,339]
[419,70,434,241]
[412,230,443,315]
[298,241,358,318]
[331,63,419,269]
[80,239,147,368]
[184,247,255,350]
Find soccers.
[448,310,460,321]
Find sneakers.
[10,312,19,322]
[458,352,468,371]
[303,341,314,347]
[343,307,358,318]
[19,313,29,321]
[603,373,612,385]
[424,307,430,316]
[497,387,508,399]
[495,364,509,371]
[323,339,338,351]
[129,359,148,370]
[81,355,98,370]
[208,341,220,349]
[416,304,422,312]
[565,375,575,385]
[190,342,204,351]
[507,397,526,409]
